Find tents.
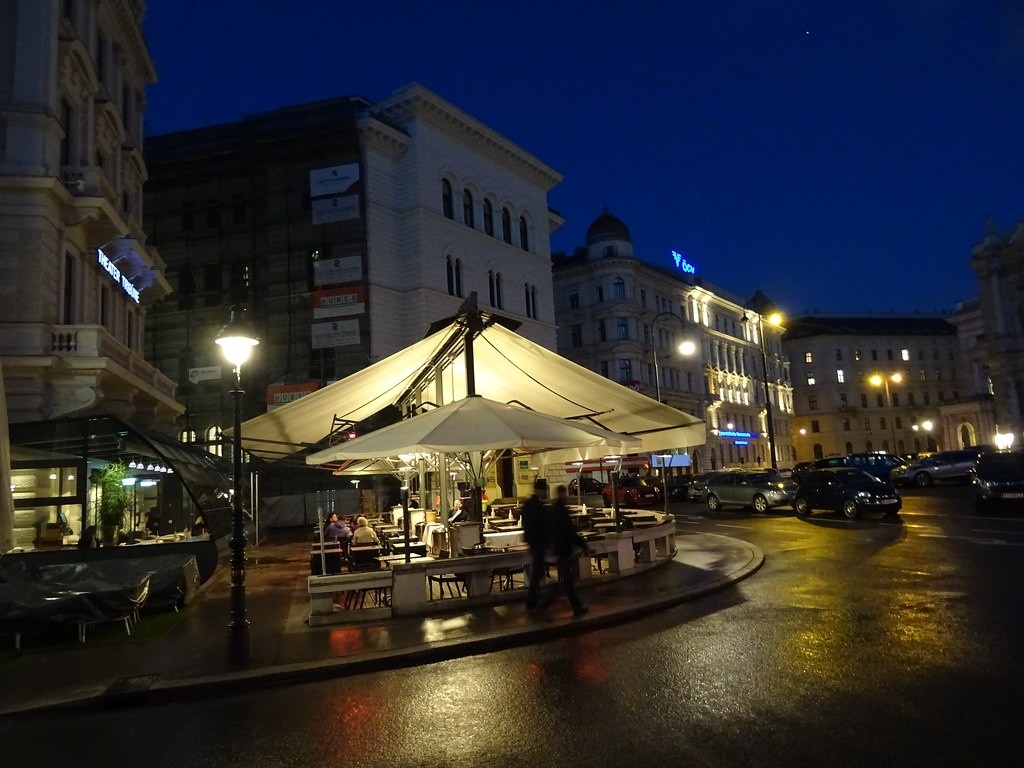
[222,315,707,549]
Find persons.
[620,472,629,477]
[741,457,744,464]
[323,513,338,531]
[351,517,378,543]
[520,479,590,619]
[325,515,350,537]
[757,456,761,463]
[409,497,418,509]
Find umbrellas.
[305,396,642,523]
[333,456,462,475]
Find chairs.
[334,505,640,610]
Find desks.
[310,503,656,571]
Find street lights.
[209,302,264,667]
[741,299,787,470]
[912,414,933,451]
[868,373,904,454]
[650,312,697,507]
[351,477,360,514]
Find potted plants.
[91,461,136,545]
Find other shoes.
[525,603,535,611]
[574,604,589,616]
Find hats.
[534,479,547,489]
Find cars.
[915,452,937,463]
[603,476,672,508]
[703,469,795,512]
[568,478,608,495]
[889,450,986,488]
[792,453,909,484]
[667,474,708,502]
[965,450,1024,510]
[789,467,904,520]
